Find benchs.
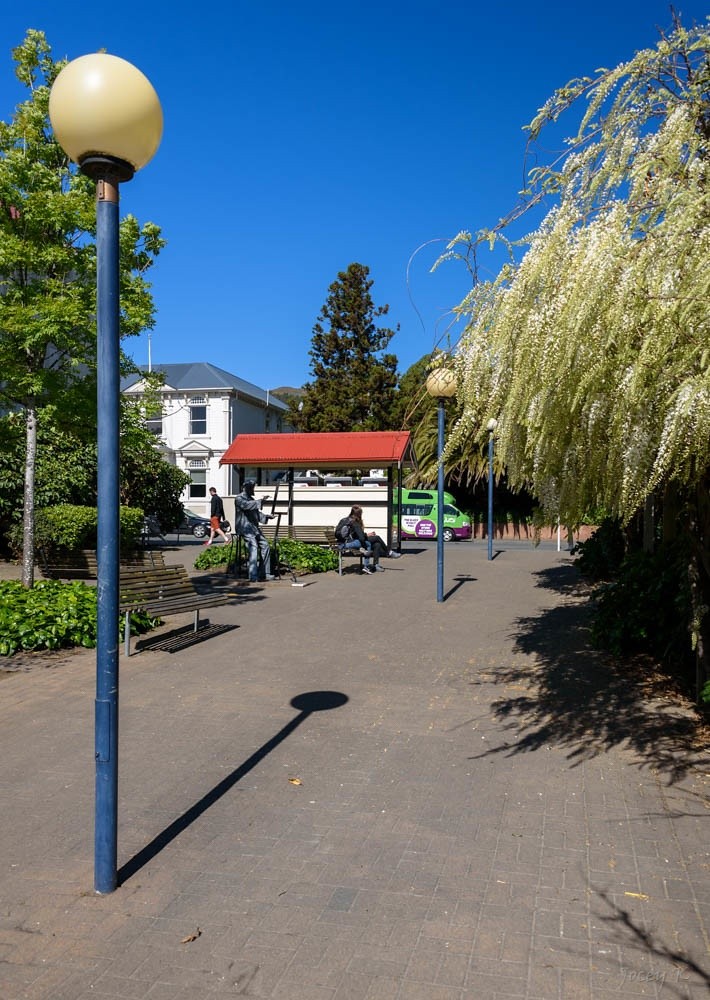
[39,547,228,657]
[258,526,364,575]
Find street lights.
[47,52,165,895]
[426,367,457,603]
[486,418,497,560]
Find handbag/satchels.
[220,520,230,532]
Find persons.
[205,487,231,544]
[235,480,275,582]
[336,506,401,573]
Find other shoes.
[389,550,401,558]
[359,548,369,556]
[376,565,386,573]
[204,542,212,547]
[363,567,373,576]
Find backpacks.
[335,518,352,540]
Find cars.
[168,509,225,539]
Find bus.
[392,487,472,542]
